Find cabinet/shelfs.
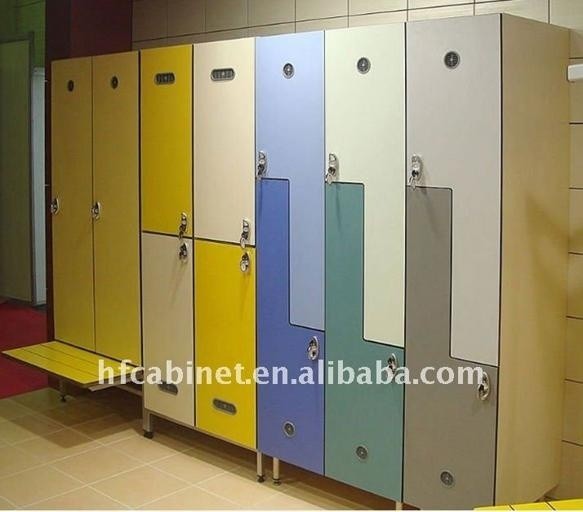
[474,499,582,511]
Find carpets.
[1,297,46,398]
